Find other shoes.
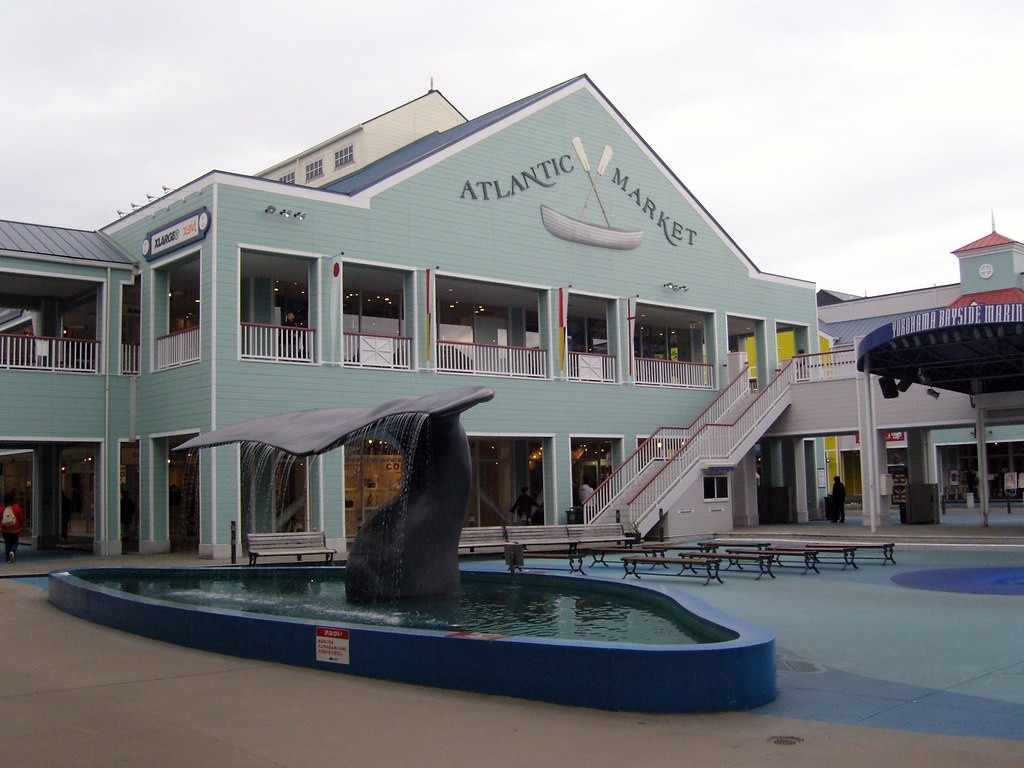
[9,551,15,561]
[839,520,844,523]
[7,560,11,564]
[831,520,837,523]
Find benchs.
[458,526,517,553]
[246,531,337,567]
[505,525,579,552]
[508,554,587,576]
[621,556,724,586]
[697,540,772,565]
[676,552,776,581]
[805,542,897,566]
[767,546,860,571]
[567,522,635,552]
[577,547,670,571]
[725,548,821,575]
[642,545,720,567]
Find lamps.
[146,194,186,203]
[926,385,940,399]
[878,375,914,398]
[280,208,292,218]
[117,209,155,218]
[294,212,306,220]
[131,203,169,212]
[162,186,202,195]
[265,205,276,214]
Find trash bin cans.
[564,507,584,524]
[825,496,840,520]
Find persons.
[832,475,846,524]
[579,482,597,507]
[169,483,181,535]
[0,487,31,563]
[795,349,810,382]
[278,313,304,361]
[61,490,71,542]
[509,487,541,522]
[121,490,135,541]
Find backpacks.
[2,506,16,527]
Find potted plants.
[625,521,641,545]
